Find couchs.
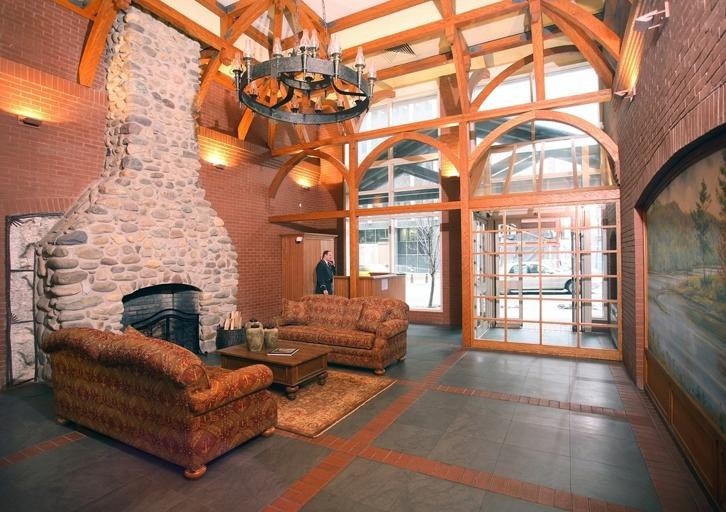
[35,323,279,483]
[274,292,410,375]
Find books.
[266,348,300,356]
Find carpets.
[275,369,398,439]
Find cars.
[477,261,582,295]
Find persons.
[316,251,337,296]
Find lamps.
[614,89,636,103]
[231,0,380,123]
[636,0,673,30]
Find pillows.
[281,298,311,326]
[358,303,397,333]
